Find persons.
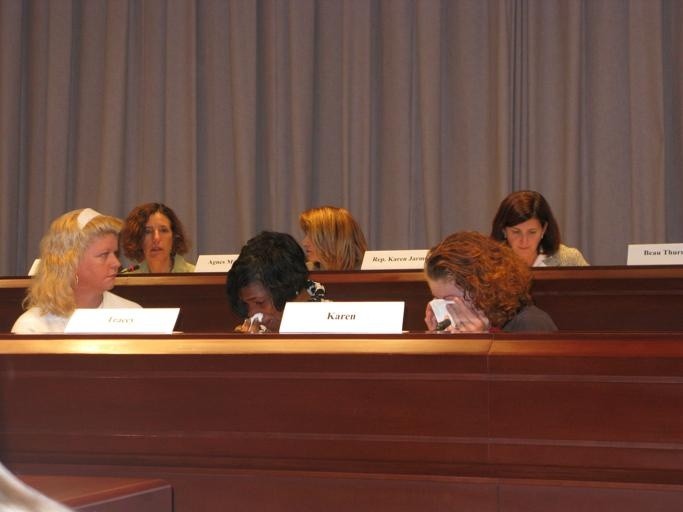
[224,229,333,334]
[487,188,594,267]
[299,204,366,271]
[9,206,144,336]
[118,201,196,273]
[422,227,560,334]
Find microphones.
[428,319,451,334]
[121,265,140,274]
